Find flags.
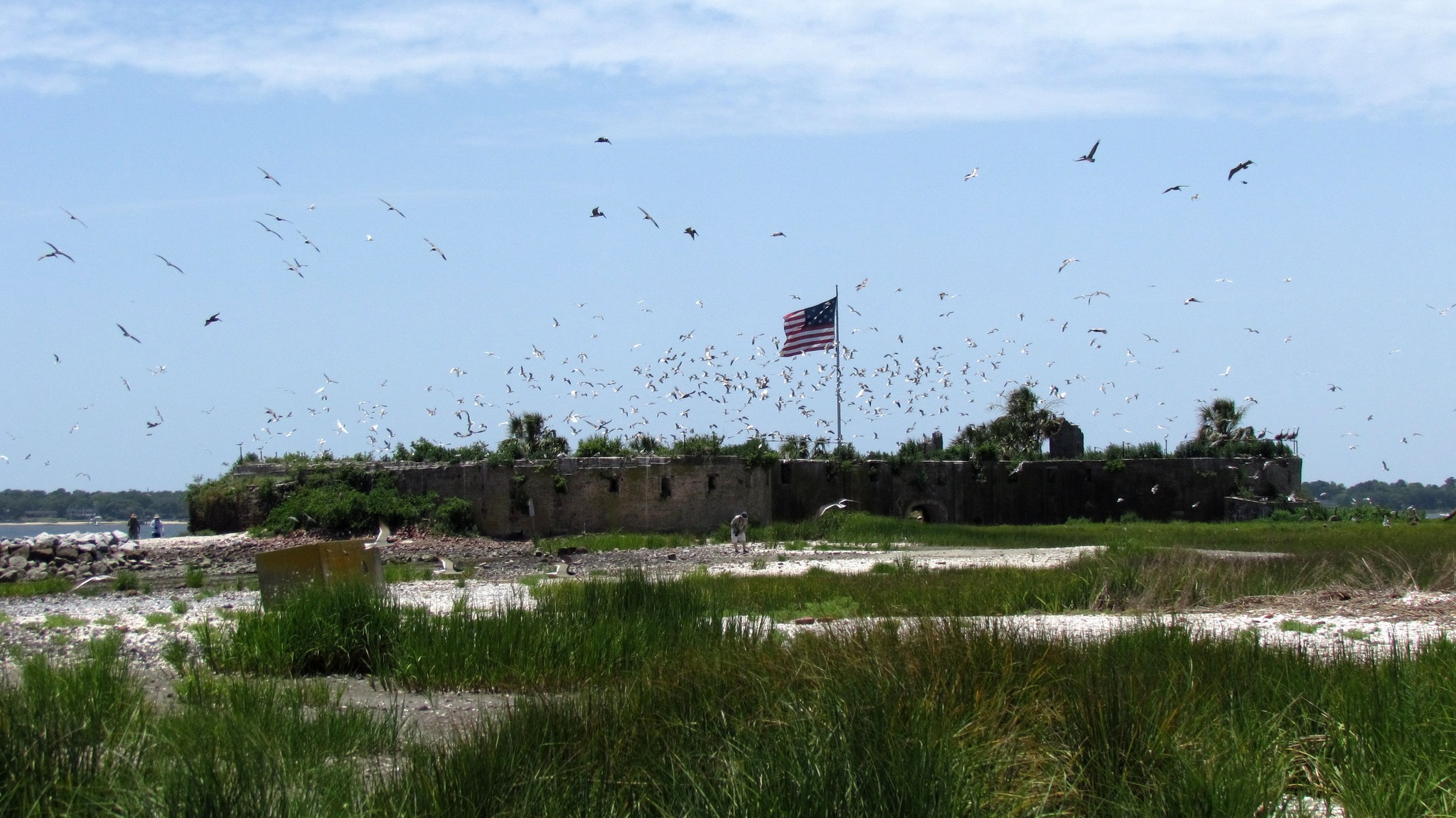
[779,296,838,357]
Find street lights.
[1164,433,1168,457]
[1125,441,1131,459]
[681,430,687,454]
[237,443,242,461]
[256,448,263,461]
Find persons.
[557,546,589,575]
[150,515,164,538]
[126,513,140,540]
[731,512,749,553]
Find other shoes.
[743,550,748,552]
[735,550,740,553]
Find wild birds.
[594,137,612,145]
[432,552,471,579]
[363,521,401,551]
[0,166,1455,528]
[1161,184,1189,193]
[815,498,861,519]
[1227,160,1258,181]
[965,167,979,181]
[1073,139,1100,162]
[544,561,583,583]
[73,575,118,591]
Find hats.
[154,515,160,518]
[132,514,137,518]
[741,512,749,518]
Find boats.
[90,517,102,526]
